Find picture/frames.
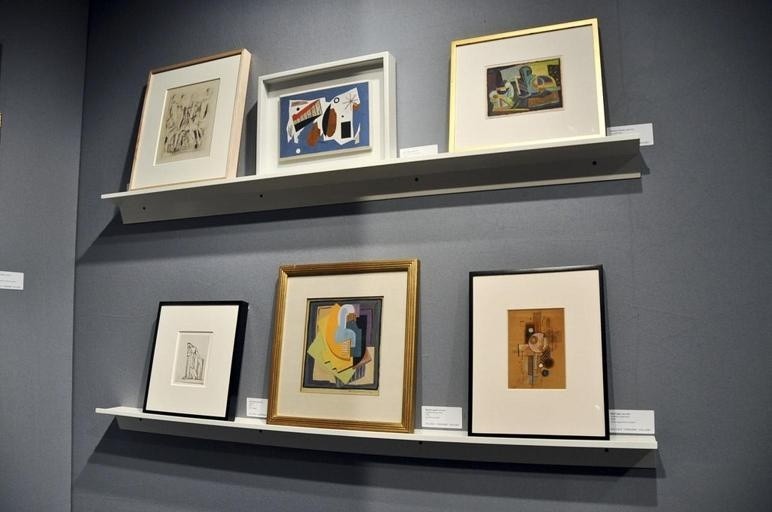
[255,51,397,177]
[266,258,420,434]
[466,263,610,439]
[448,17,606,158]
[143,301,249,420]
[125,47,252,193]
